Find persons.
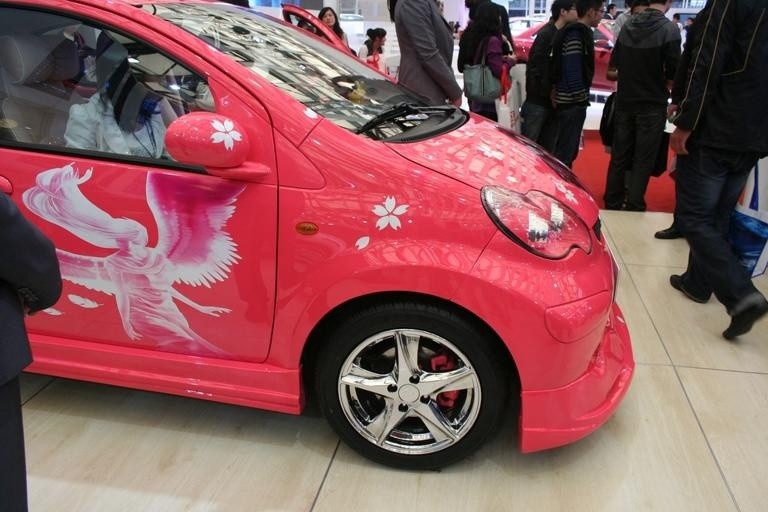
[319,7,348,48]
[0,194,63,512]
[357,28,386,76]
[388,0,463,108]
[64,25,180,164]
[458,0,768,339]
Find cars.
[0,0,636,471]
[613,10,624,20]
[509,19,545,35]
[512,20,617,92]
[665,8,701,25]
[530,14,549,18]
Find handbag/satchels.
[494,80,523,135]
[732,203,767,277]
[463,59,512,104]
[650,132,670,177]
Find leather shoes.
[723,302,768,338]
[670,275,682,290]
[655,224,683,239]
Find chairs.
[0,32,92,147]
